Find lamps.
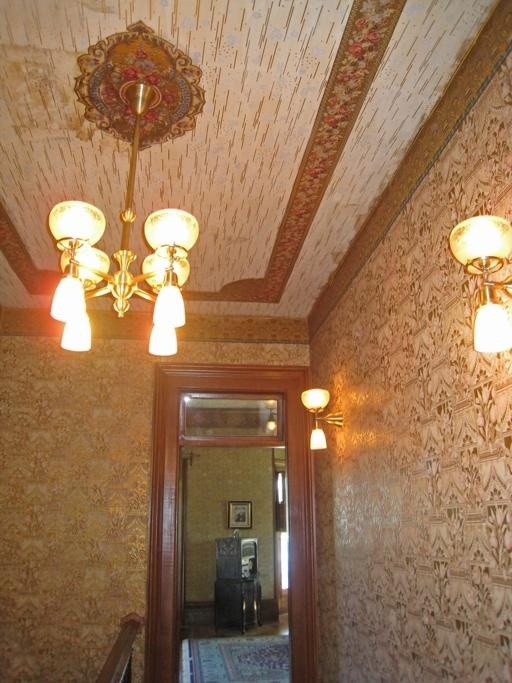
[449,215,512,351]
[47,80,200,356]
[300,388,344,450]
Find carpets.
[181,634,292,682]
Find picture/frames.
[228,500,252,528]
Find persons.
[235,507,245,522]
